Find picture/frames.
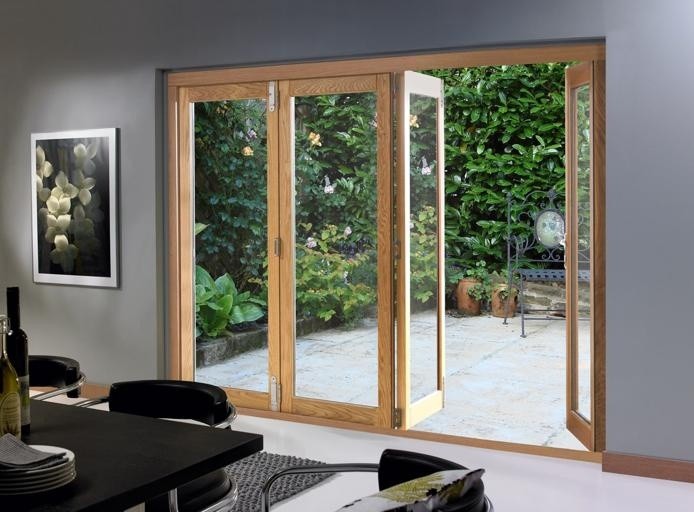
[29,126,123,290]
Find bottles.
[5,286,30,437]
[0,314,20,441]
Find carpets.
[223,451,336,512]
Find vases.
[455,277,484,316]
[492,283,517,318]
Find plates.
[0,444,75,498]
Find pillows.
[336,468,486,512]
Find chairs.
[29,353,87,403]
[502,187,593,338]
[260,448,495,512]
[70,379,240,512]
